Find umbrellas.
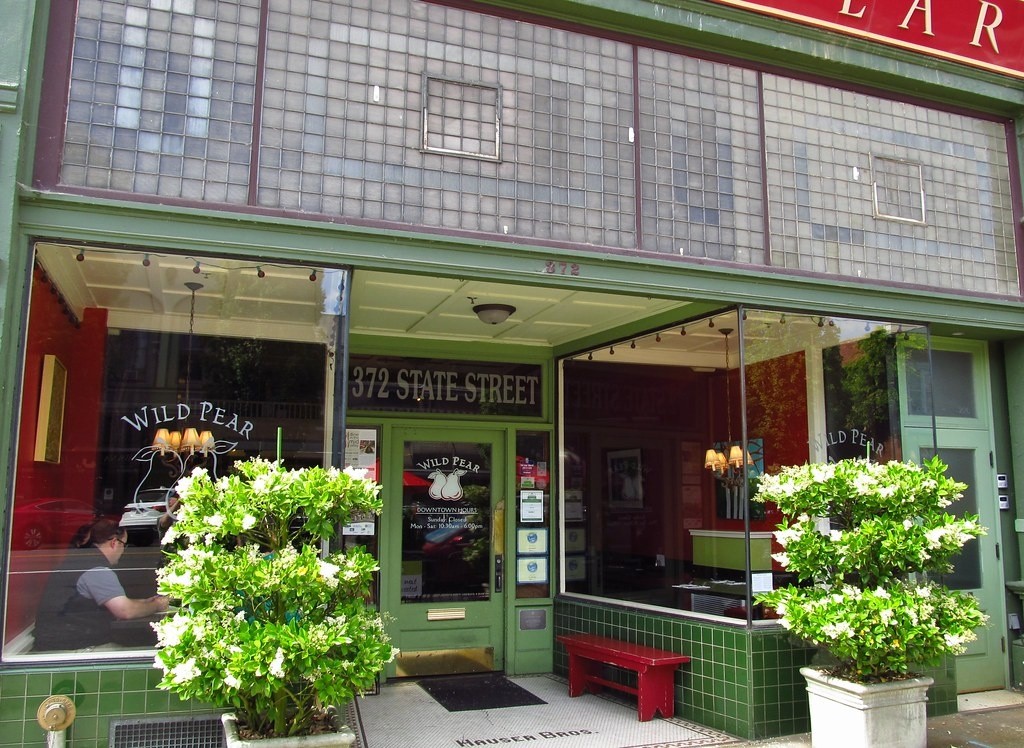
[357,461,432,487]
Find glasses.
[107,538,127,551]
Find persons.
[33,519,171,651]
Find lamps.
[705,328,754,471]
[473,304,516,325]
[152,282,215,457]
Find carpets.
[414,672,548,712]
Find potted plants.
[150,428,399,748]
[750,441,990,748]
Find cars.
[10,496,121,549]
[117,484,182,547]
[422,512,489,589]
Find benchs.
[556,633,691,721]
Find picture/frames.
[600,447,645,512]
[712,438,767,520]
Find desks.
[672,578,747,617]
[110,614,174,629]
